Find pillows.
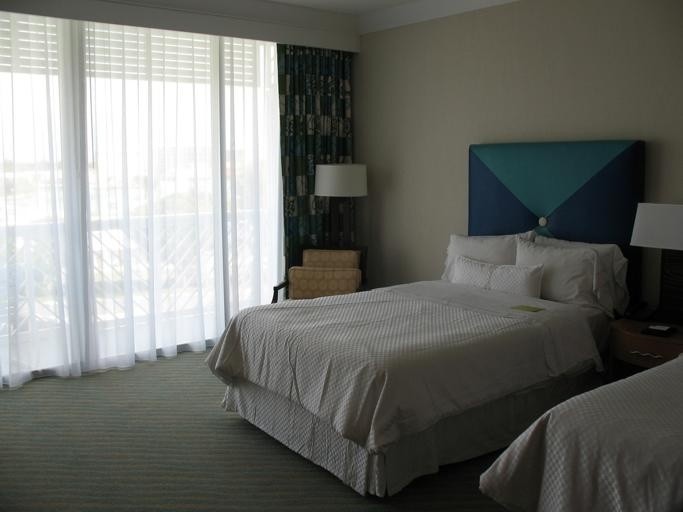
[438,229,630,317]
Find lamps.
[313,163,368,248]
[626,203,683,325]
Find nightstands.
[609,316,683,373]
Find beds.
[515,353,682,512]
[227,141,646,503]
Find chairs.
[271,248,364,302]
